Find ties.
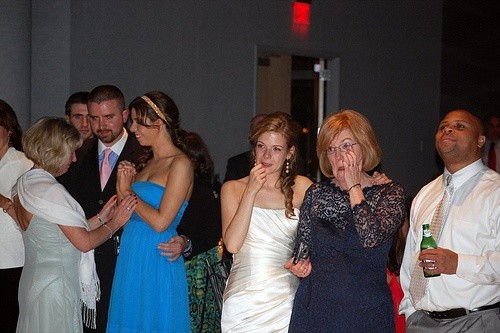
[408,175,454,303]
[99,149,112,192]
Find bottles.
[420,224,440,278]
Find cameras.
[293,238,311,264]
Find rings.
[432,262,434,268]
[127,207,130,210]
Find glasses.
[325,141,360,155]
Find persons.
[10,116,138,333]
[0,91,500,333]
[287,110,406,333]
[105,91,214,333]
[399,110,500,333]
[219,112,392,333]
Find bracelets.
[347,183,361,192]
[97,214,104,225]
[3,200,13,213]
[103,224,112,238]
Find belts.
[426,301,500,319]
